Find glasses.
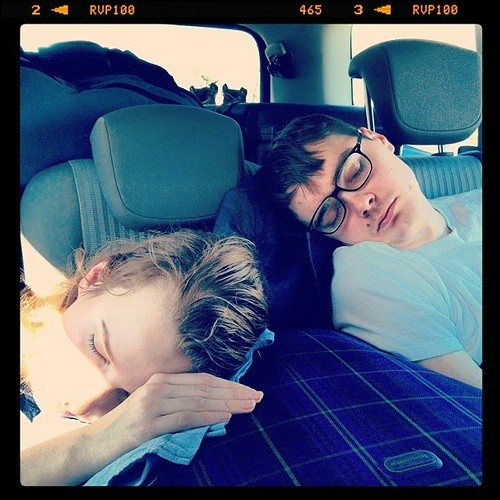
[307,128,373,235]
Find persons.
[261,113,482,392]
[21,224,268,487]
